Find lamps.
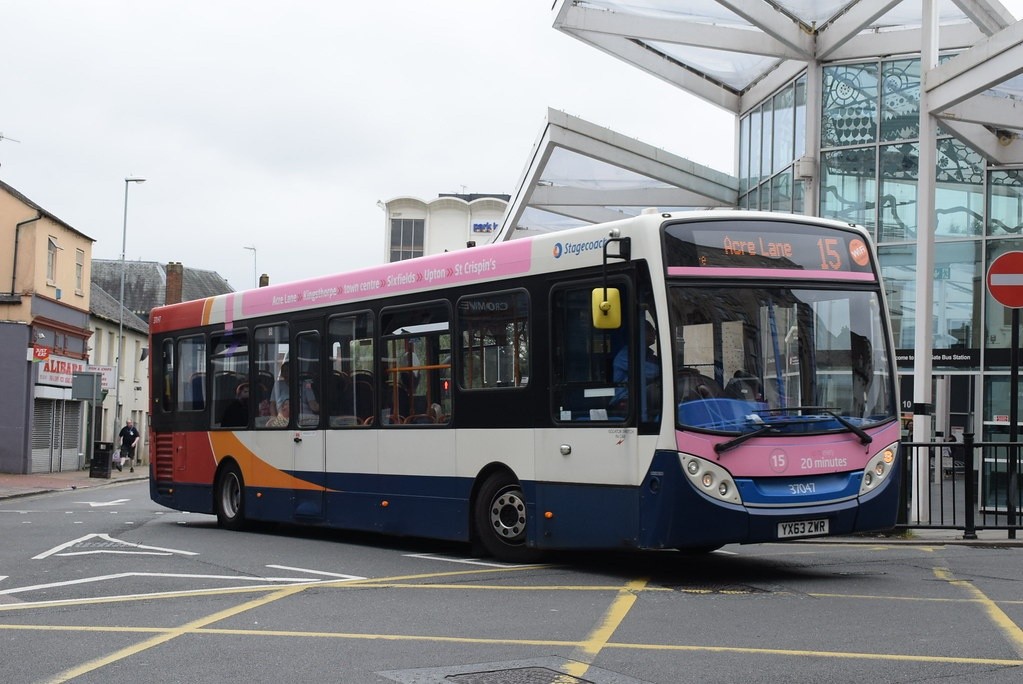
[38,334,46,340]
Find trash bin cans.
[89,441,114,479]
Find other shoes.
[116,464,122,471]
[131,468,134,472]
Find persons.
[116,419,140,473]
[608,321,661,407]
[221,362,319,428]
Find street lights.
[113,176,147,469]
[245,246,259,291]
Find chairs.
[191,366,418,425]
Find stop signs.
[986,250,1023,309]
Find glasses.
[241,391,249,393]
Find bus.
[147,206,903,563]
